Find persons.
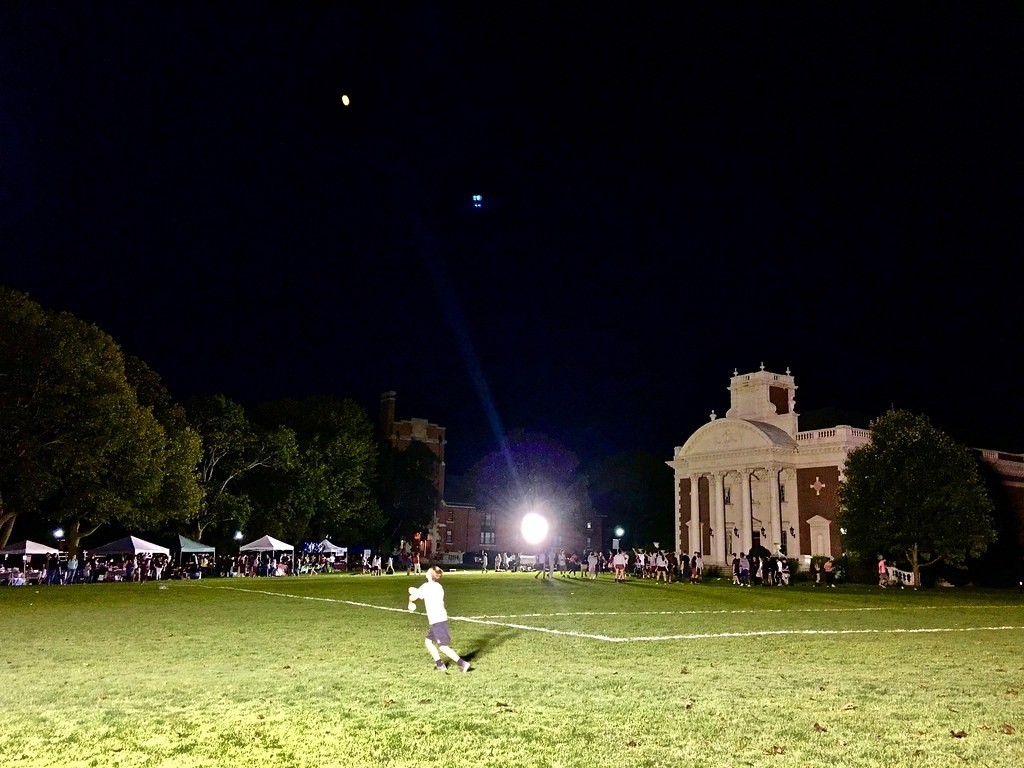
[408,566,471,674]
[877,559,889,588]
[475,547,704,586]
[731,552,791,588]
[823,557,836,588]
[812,557,823,588]
[0,527,466,588]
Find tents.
[155,534,216,567]
[299,539,349,573]
[85,535,170,567]
[0,537,63,585]
[239,534,294,577]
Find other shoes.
[657,583,659,584]
[462,663,470,672]
[664,583,667,585]
[691,582,700,584]
[614,577,626,584]
[535,574,598,581]
[437,664,447,671]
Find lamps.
[734,526,739,538]
[708,527,714,538]
[789,526,796,539]
[760,526,766,538]
